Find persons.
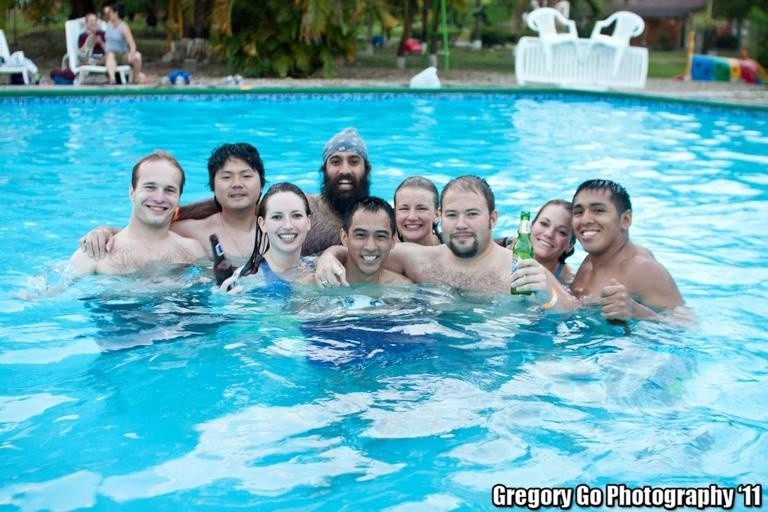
[498,201,575,312]
[101,5,146,86]
[21,152,206,301]
[172,129,371,256]
[395,176,441,249]
[220,182,317,299]
[571,180,683,324]
[78,142,265,280]
[317,174,581,316]
[74,14,106,67]
[299,197,416,291]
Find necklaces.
[218,211,254,263]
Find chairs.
[583,11,643,76]
[0,29,29,85]
[65,17,132,85]
[525,7,582,73]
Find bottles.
[511,211,534,296]
[209,234,234,292]
[607,319,631,338]
